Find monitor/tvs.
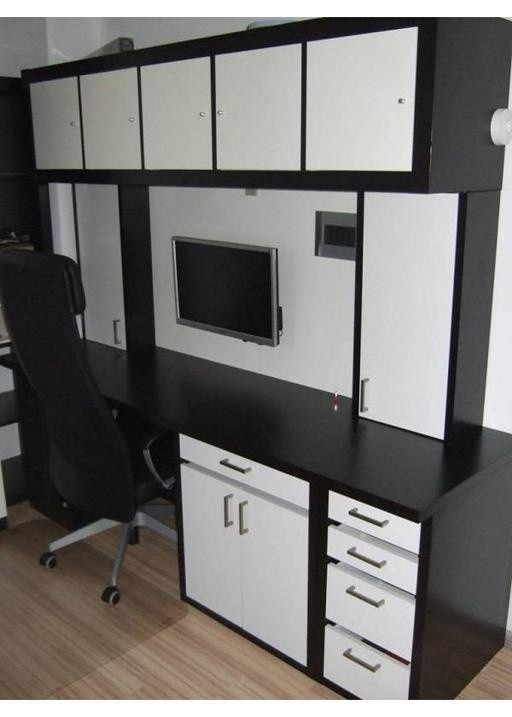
[171,235,283,347]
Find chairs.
[1,252,177,607]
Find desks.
[1,339,512,699]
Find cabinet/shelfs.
[27,73,87,171]
[77,65,143,173]
[306,22,420,174]
[177,429,310,667]
[44,182,129,352]
[323,490,420,699]
[140,42,304,174]
[357,189,462,442]
